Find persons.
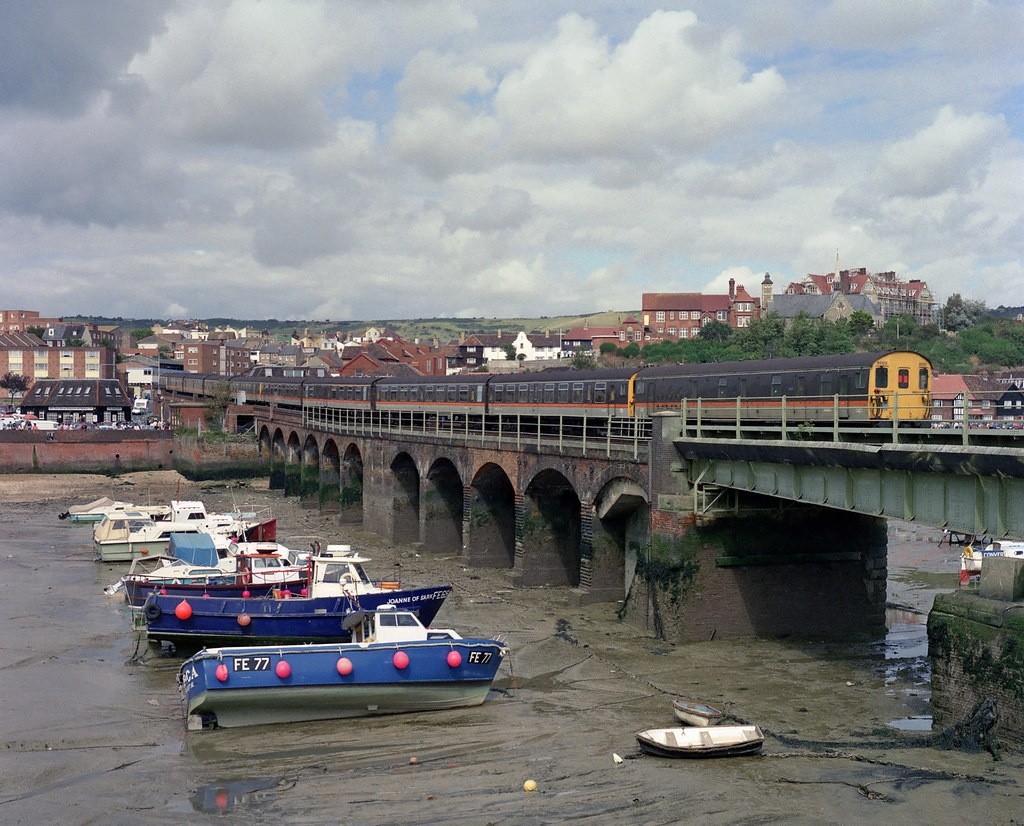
[0,392,170,430]
[930,417,1024,428]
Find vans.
[133,397,150,415]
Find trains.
[158,350,934,443]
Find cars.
[0,416,169,432]
[930,421,1024,429]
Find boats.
[958,541,1024,587]
[634,724,768,761]
[59,483,512,732]
[672,698,724,730]
